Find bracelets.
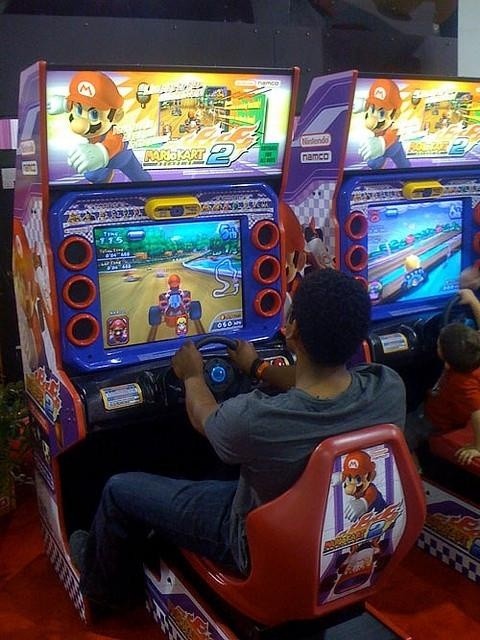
[256,360,271,381]
[249,358,262,377]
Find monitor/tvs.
[89,212,246,352]
[364,185,473,324]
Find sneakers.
[69,530,95,571]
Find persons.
[456,259,479,292]
[64,266,409,618]
[406,285,480,479]
